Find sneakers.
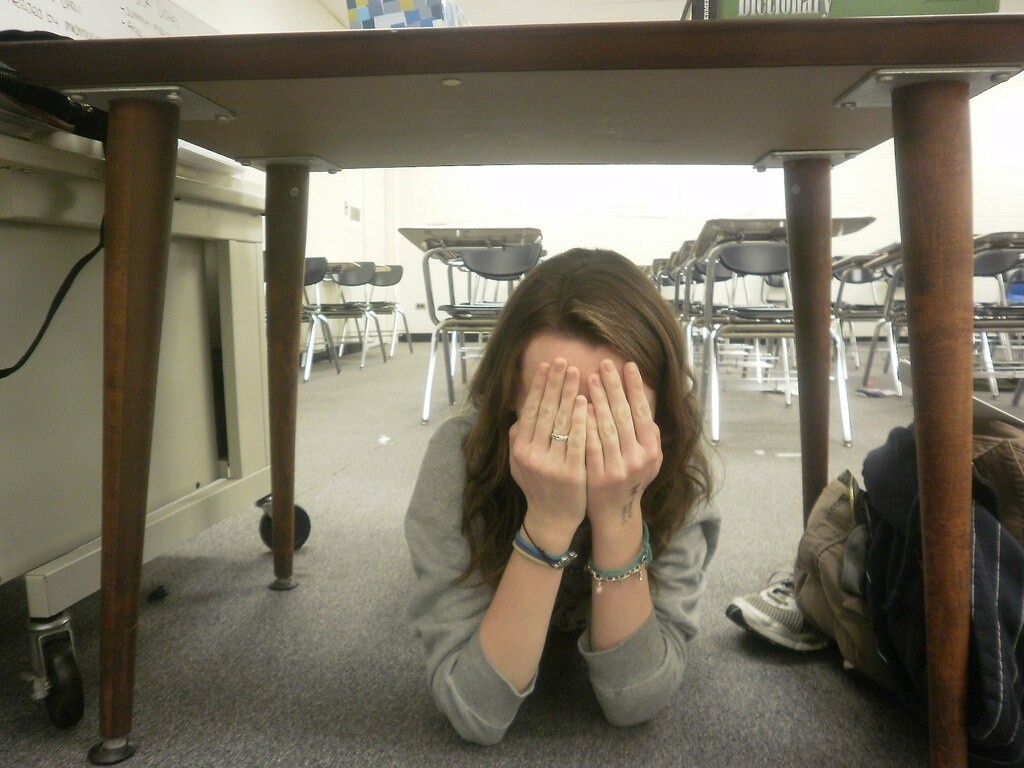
[725,566,866,678]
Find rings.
[550,431,570,442]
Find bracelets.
[588,524,653,594]
[510,520,579,574]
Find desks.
[673,240,695,271]
[973,232,1024,254]
[398,228,546,259]
[688,217,877,260]
[666,252,677,280]
[863,250,904,271]
[652,258,668,280]
[832,254,879,273]
[0,14,1024,768]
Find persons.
[727,419,1023,655]
[404,244,724,745]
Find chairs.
[656,241,852,448]
[833,248,1024,411]
[300,257,413,383]
[421,244,542,425]
